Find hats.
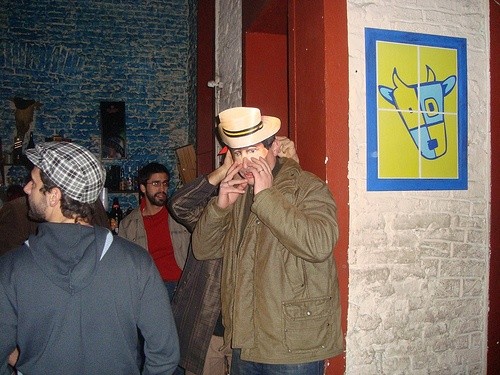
[25,141,106,204]
[218,106,281,148]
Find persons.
[192,107,345,375]
[0,141,181,375]
[0,136,300,375]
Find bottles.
[108,197,124,235]
[3,178,27,198]
[118,165,142,193]
[25,132,34,162]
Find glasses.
[232,145,271,159]
[144,182,170,186]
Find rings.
[258,169,264,173]
[227,181,231,187]
